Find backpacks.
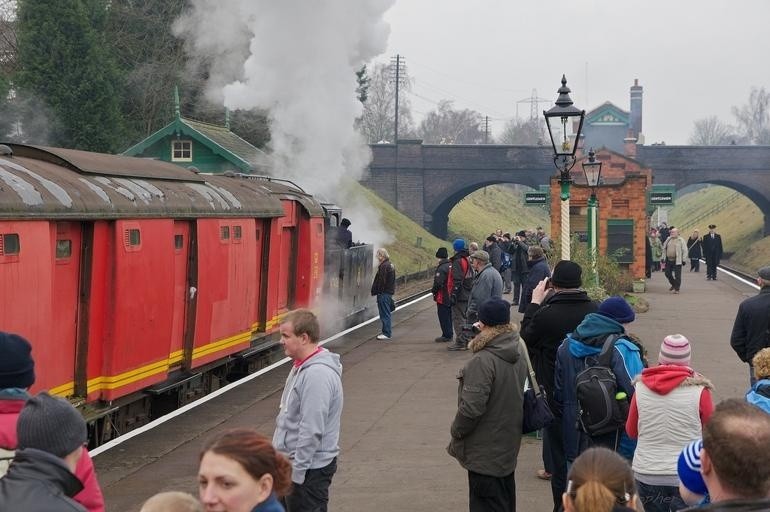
[463,256,476,289]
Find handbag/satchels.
[575,367,630,437]
[522,385,555,434]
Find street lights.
[582,148,603,287]
[543,73,586,265]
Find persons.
[647,221,724,293]
[0,391,91,512]
[730,266,770,386]
[552,296,647,512]
[198,428,288,512]
[675,397,770,511]
[561,445,645,512]
[271,307,344,511]
[370,247,397,340]
[676,437,711,507]
[330,217,352,248]
[443,294,529,511]
[625,334,717,511]
[430,216,558,352]
[518,260,600,481]
[744,345,770,412]
[0,330,107,512]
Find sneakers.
[435,337,453,342]
[448,343,466,351]
[537,469,552,480]
[377,334,392,340]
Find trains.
[0,139,374,455]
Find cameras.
[546,278,554,289]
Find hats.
[16,393,88,458]
[709,225,716,229]
[436,248,448,258]
[0,332,34,388]
[471,249,489,261]
[342,218,351,226]
[658,334,691,365]
[453,239,464,251]
[596,297,635,323]
[758,267,770,280]
[678,439,708,495]
[552,261,582,288]
[478,300,510,326]
